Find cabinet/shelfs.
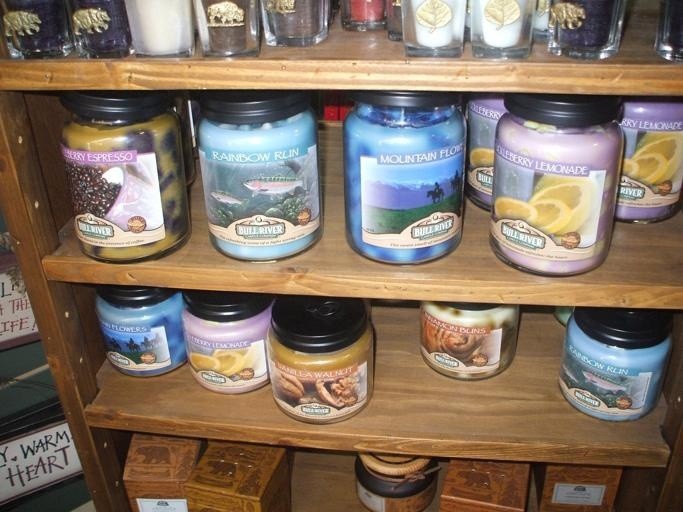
[2,6,683,502]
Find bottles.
[615,98,683,223]
[265,297,377,426]
[417,300,518,384]
[343,91,469,267]
[92,283,189,378]
[196,92,323,262]
[52,92,192,267]
[466,96,507,213]
[353,453,442,512]
[552,306,674,424]
[486,93,627,278]
[181,291,271,396]
